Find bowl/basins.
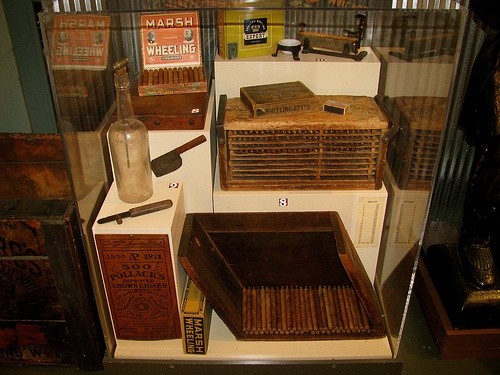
[277,38,301,55]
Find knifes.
[151,134,207,179]
[97,199,173,225]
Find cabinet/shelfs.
[0,132,107,375]
[32,1,468,375]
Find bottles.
[109,58,154,202]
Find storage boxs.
[240,81,320,119]
[179,274,213,355]
[218,8,287,60]
[323,99,351,116]
